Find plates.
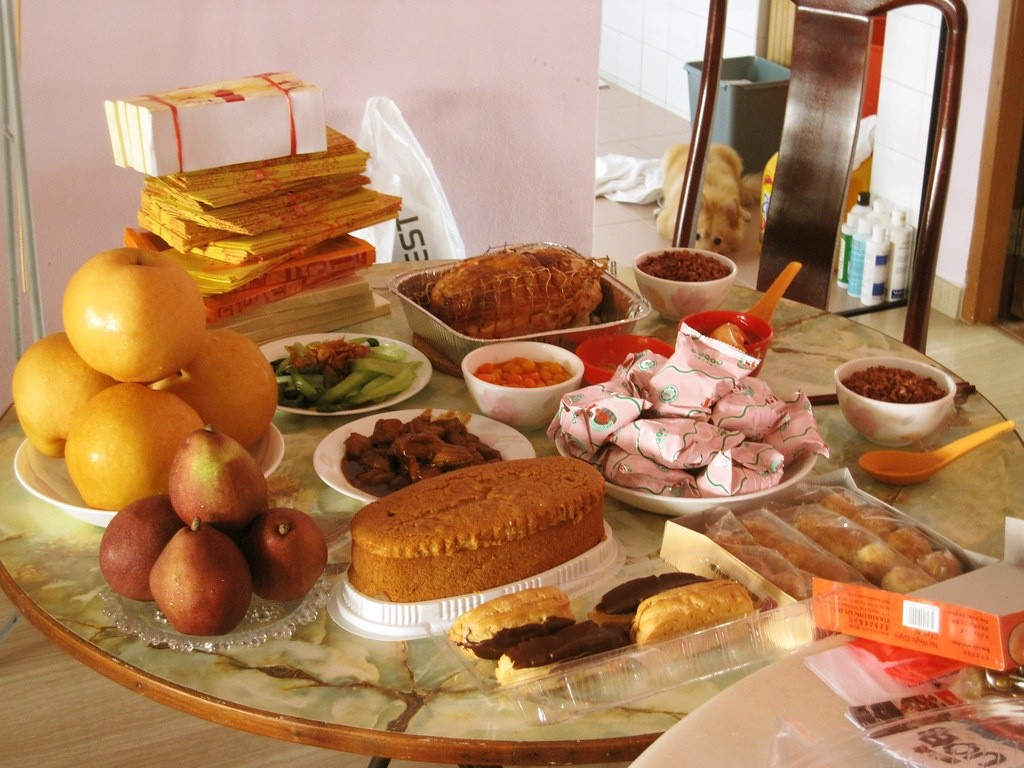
[259,333,432,416]
[313,409,537,502]
[96,563,336,652]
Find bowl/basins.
[834,356,956,448]
[677,310,774,377]
[461,342,584,431]
[555,428,819,517]
[14,423,286,527]
[387,242,653,368]
[577,333,675,386]
[634,248,738,321]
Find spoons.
[742,262,802,322]
[859,420,1016,486]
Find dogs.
[655,142,763,255]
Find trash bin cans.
[682,54,792,175]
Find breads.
[346,455,606,602]
[427,245,611,340]
[442,570,755,688]
[704,489,966,607]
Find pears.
[98,424,328,638]
[10,246,280,510]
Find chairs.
[672,0,970,356]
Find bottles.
[758,151,779,251]
[837,191,917,307]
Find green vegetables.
[266,336,424,412]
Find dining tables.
[0,258,1024,768]
[629,631,1024,768]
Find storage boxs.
[658,469,1024,681]
[388,242,651,367]
